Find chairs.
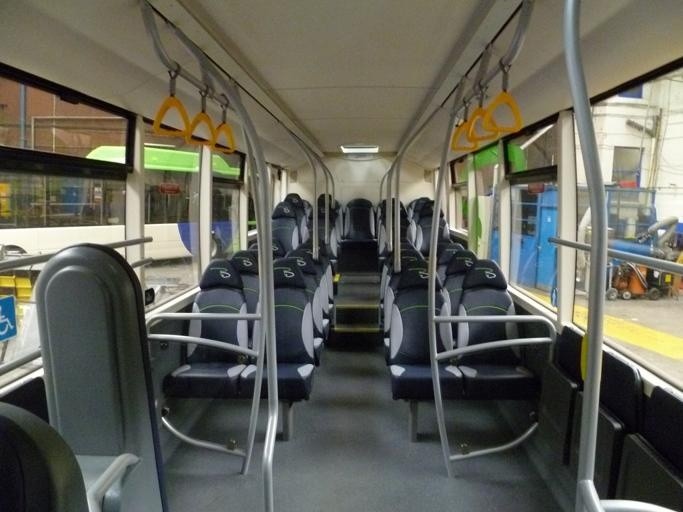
[163,194,534,442]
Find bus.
[1,140,256,367]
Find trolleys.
[584,259,620,301]
